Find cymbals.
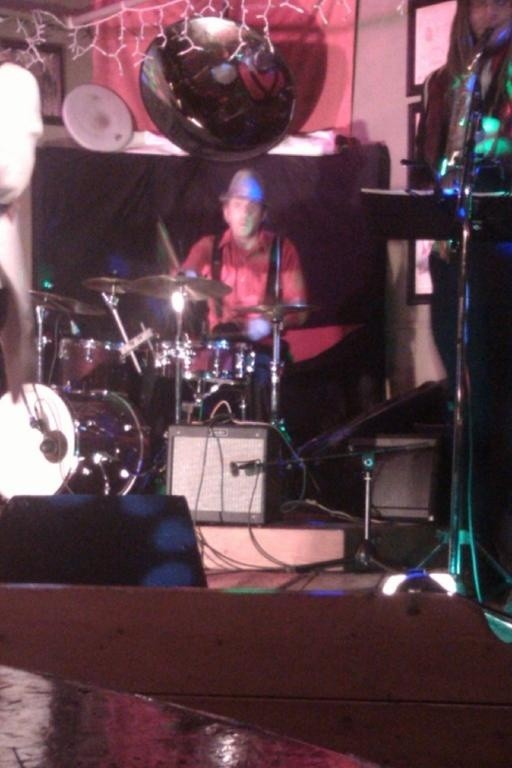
[120,275,234,303]
[80,276,134,294]
[229,301,324,319]
[23,286,107,318]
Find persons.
[173,168,308,336]
[409,0,512,379]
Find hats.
[219,167,274,208]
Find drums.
[181,343,254,386]
[150,342,183,380]
[0,384,152,504]
[57,339,154,408]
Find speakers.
[166,423,282,527]
[340,435,452,526]
[2,492,208,591]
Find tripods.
[415,57,512,588]
[285,456,413,578]
